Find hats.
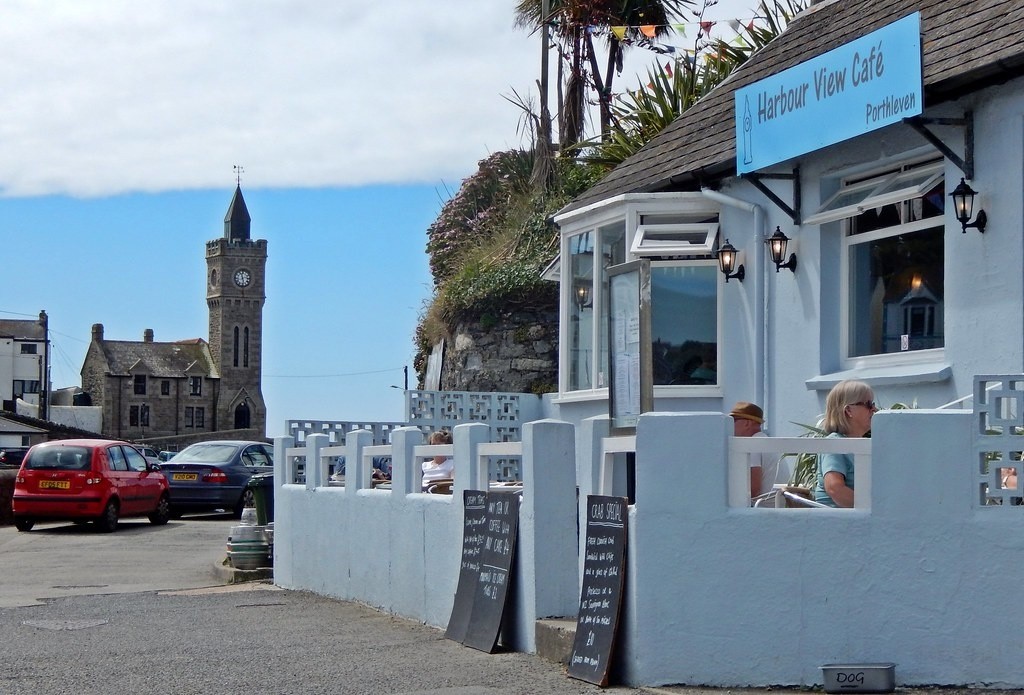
[728,403,766,424]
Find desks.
[326,473,523,498]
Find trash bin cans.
[247,471,274,526]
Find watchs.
[1003,474,1015,488]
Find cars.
[241,470,274,514]
[158,440,274,519]
[12,439,172,534]
[129,444,179,471]
[0,445,28,526]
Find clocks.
[233,268,253,288]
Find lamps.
[762,226,798,274]
[948,177,988,234]
[714,239,755,283]
[574,281,593,310]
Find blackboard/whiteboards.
[443,490,488,645]
[567,494,628,688]
[462,491,520,655]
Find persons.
[333,456,392,480]
[728,402,790,507]
[815,380,878,508]
[986,451,1024,505]
[422,429,454,486]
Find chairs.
[60,451,76,465]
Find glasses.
[847,401,876,414]
[733,417,748,422]
